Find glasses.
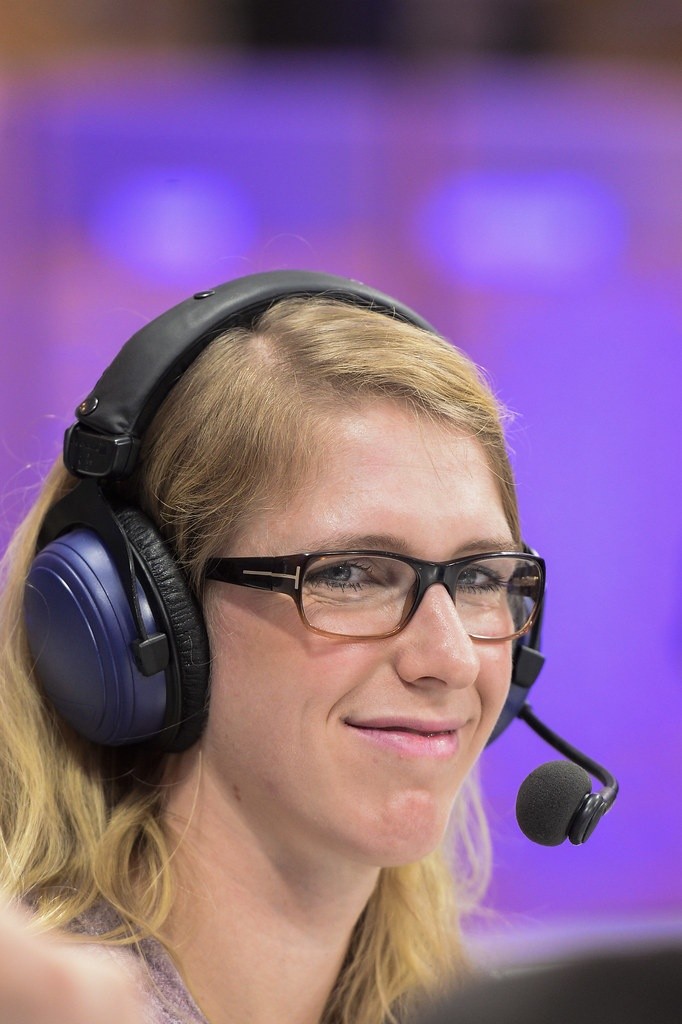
[203,541,546,642]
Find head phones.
[28,269,547,750]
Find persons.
[1,268,546,1023]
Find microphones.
[514,703,617,845]
